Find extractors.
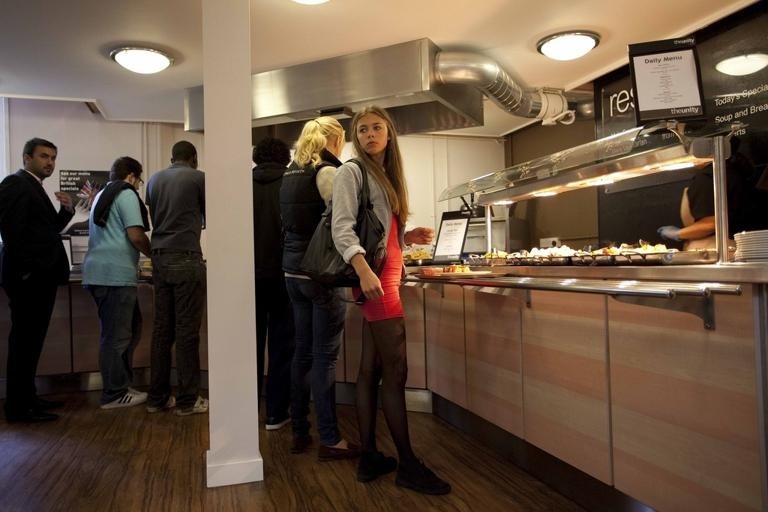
[183,37,484,150]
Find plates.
[439,271,492,277]
[733,230,768,261]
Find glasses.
[138,177,144,186]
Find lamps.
[537,29,600,62]
[716,54,768,76]
[109,46,174,76]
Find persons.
[253,136,293,432]
[80,158,152,410]
[60,199,91,234]
[278,116,360,463]
[330,105,452,496]
[656,132,740,250]
[145,140,209,418]
[1,136,75,424]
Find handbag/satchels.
[300,159,389,304]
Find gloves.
[657,225,681,242]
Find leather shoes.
[290,435,312,454]
[6,405,59,424]
[318,443,358,462]
[24,397,64,409]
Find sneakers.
[266,411,291,430]
[172,395,209,416]
[395,457,451,495]
[145,395,176,412]
[99,387,148,409]
[357,451,397,482]
